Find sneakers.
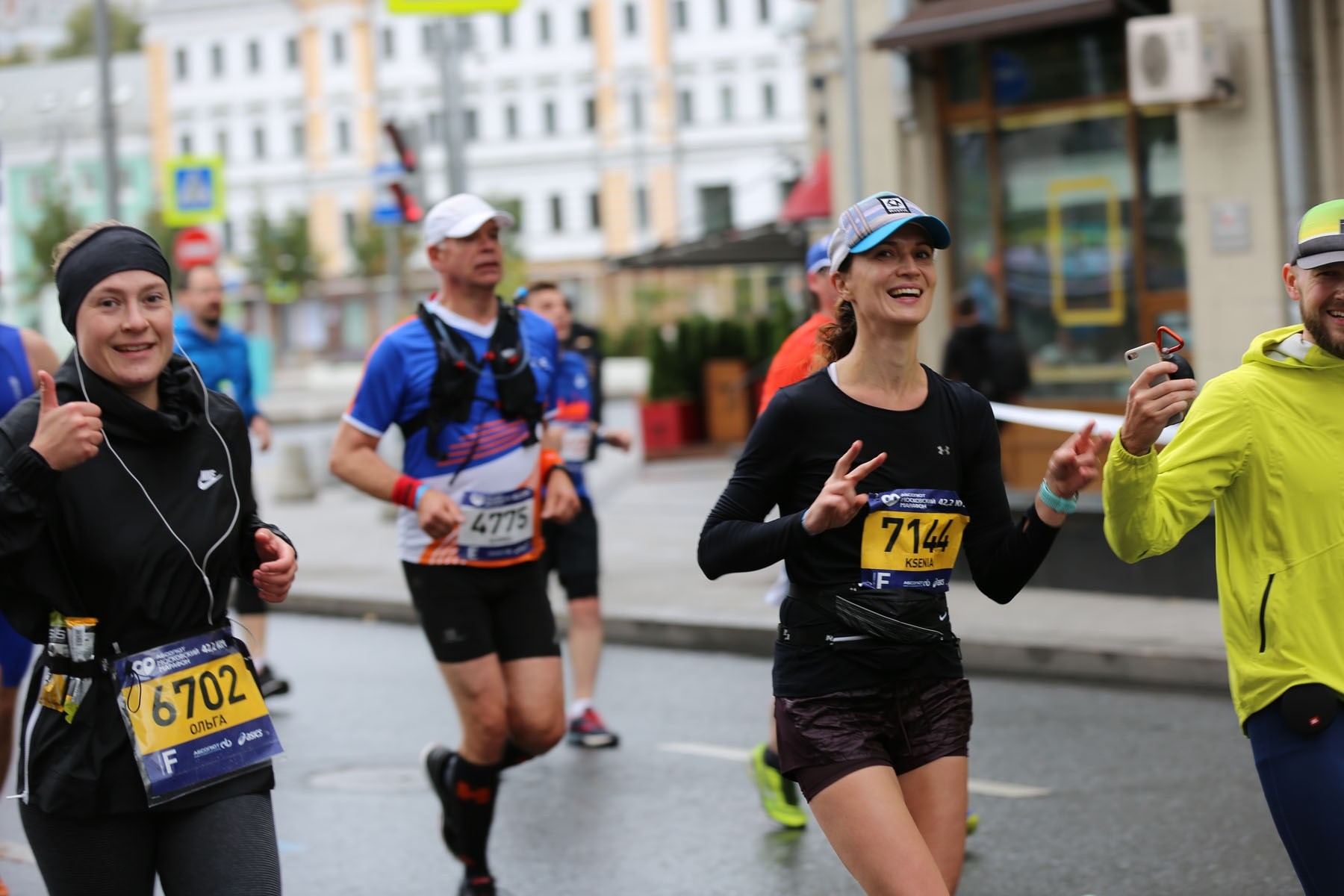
[565,706,618,748]
[749,746,808,827]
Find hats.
[805,234,832,275]
[1290,198,1344,270]
[826,191,951,275]
[422,193,516,248]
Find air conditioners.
[1123,9,1241,108]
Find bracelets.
[1038,478,1076,513]
[540,449,575,485]
[391,476,430,509]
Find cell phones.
[1123,343,1185,429]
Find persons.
[698,193,1115,896]
[513,282,631,747]
[331,193,576,895]
[0,221,298,896]
[1101,199,1344,896]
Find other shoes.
[457,871,498,896]
[257,665,289,699]
[426,745,479,867]
